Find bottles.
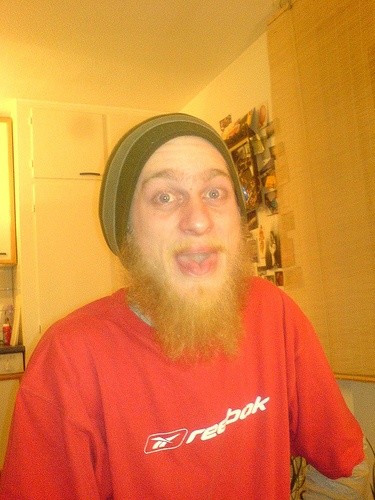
[3,324,10,345]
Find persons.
[0,113,369,500]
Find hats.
[100,114,245,255]
